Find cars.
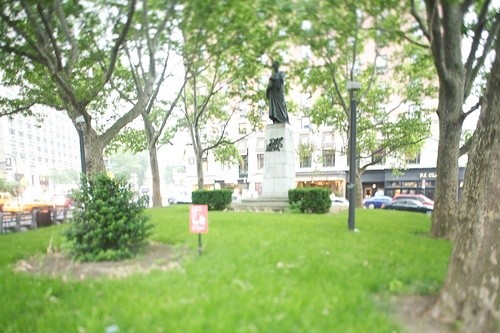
[2,196,55,213]
[380,198,435,216]
[364,195,392,208]
[329,198,350,209]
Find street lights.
[75,115,88,182]
[344,80,362,228]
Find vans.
[391,194,434,208]
